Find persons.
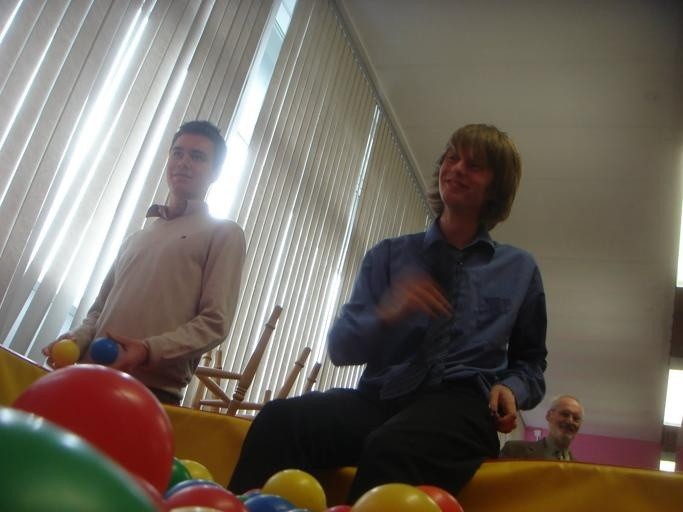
[41,120,246,406]
[227,124,550,500]
[500,395,583,460]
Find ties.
[377,245,461,403]
